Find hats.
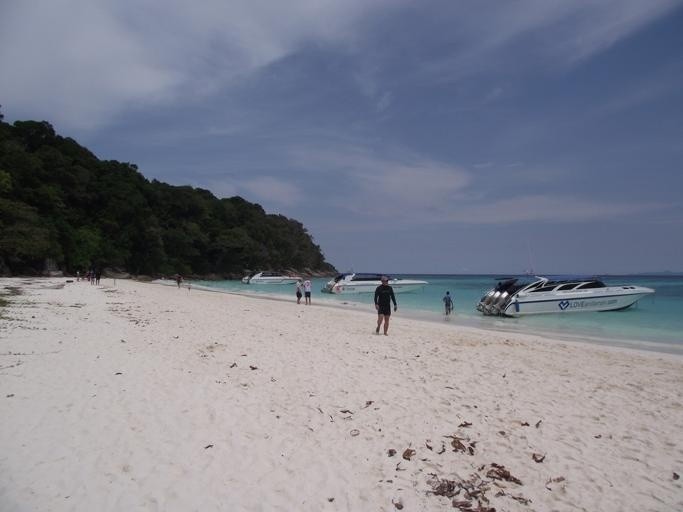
[380,276,388,282]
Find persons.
[304,279,313,304]
[296,278,303,304]
[374,275,397,336]
[176,272,182,288]
[77,269,101,285]
[443,292,453,315]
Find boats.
[322,272,431,298]
[242,267,303,287]
[473,270,657,316]
